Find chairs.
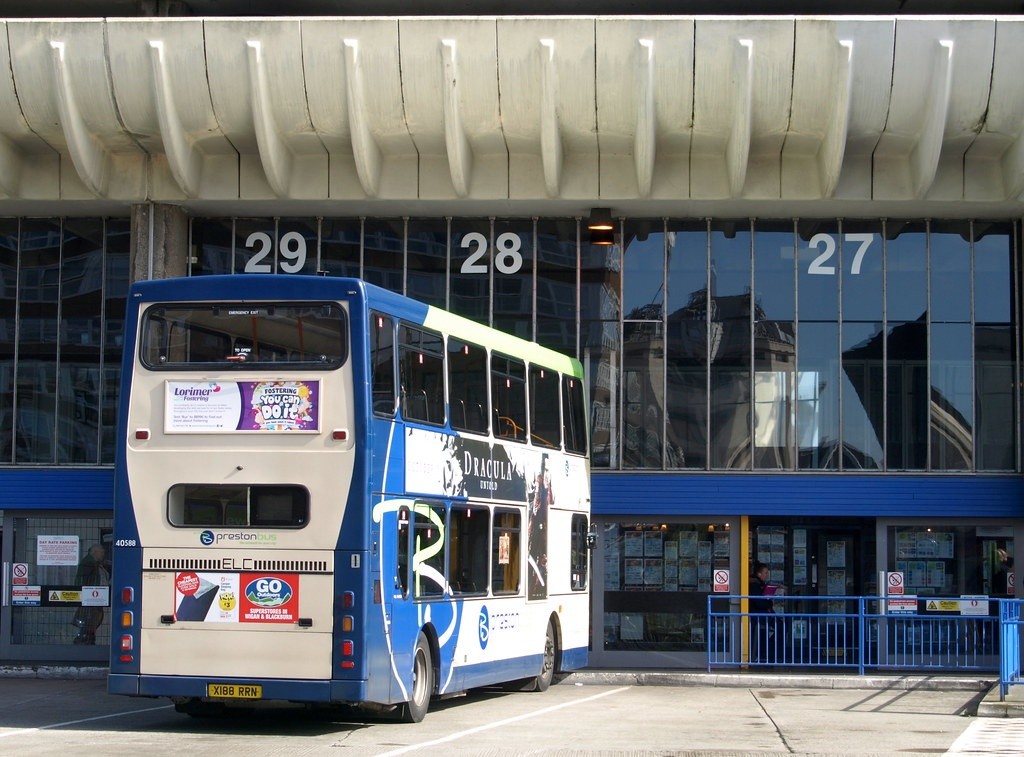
[373,383,504,436]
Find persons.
[73,543,110,644]
[749,560,773,668]
[974,548,1014,653]
[527,453,555,600]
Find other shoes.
[74,638,93,645]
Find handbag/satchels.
[72,606,103,634]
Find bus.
[109,273,593,729]
